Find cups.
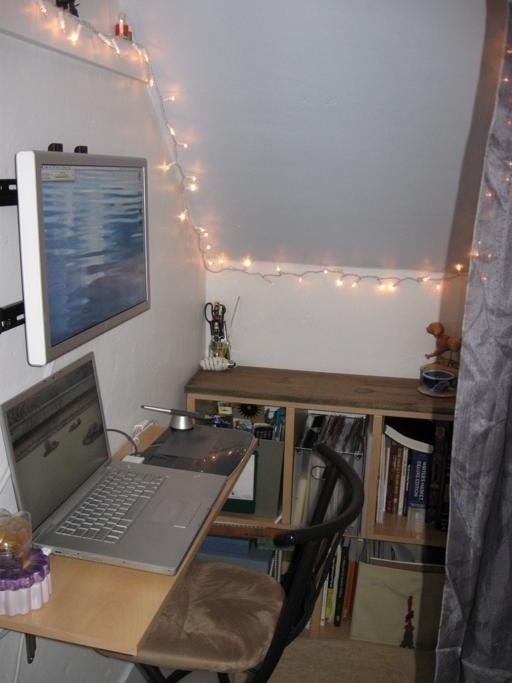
[421,369,456,394]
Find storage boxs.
[350,542,446,650]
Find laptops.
[0,353,229,578]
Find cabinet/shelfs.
[185,365,455,640]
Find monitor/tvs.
[13,151,151,368]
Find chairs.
[96,444,365,682]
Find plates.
[416,385,456,398]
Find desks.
[0,423,257,656]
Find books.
[283,409,454,628]
